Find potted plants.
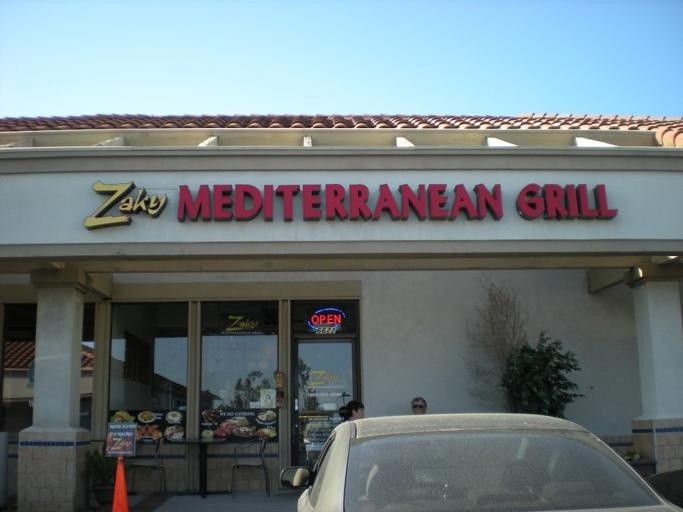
[83,446,117,506]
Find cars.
[280,413,683,511]
[297,419,337,463]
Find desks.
[174,437,228,499]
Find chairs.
[229,434,271,501]
[126,435,168,496]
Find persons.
[340,399,386,424]
[409,397,427,415]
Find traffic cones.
[110,456,130,511]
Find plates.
[137,411,156,424]
[165,411,183,424]
[137,429,162,441]
[258,427,277,439]
[253,415,277,424]
[164,425,184,440]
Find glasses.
[413,404,423,409]
